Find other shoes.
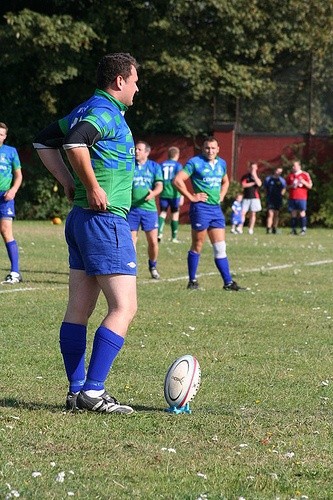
[236,226,243,235]
[170,238,180,244]
[287,228,296,236]
[230,229,238,236]
[246,229,254,235]
[298,230,308,237]
[156,233,163,243]
[149,265,163,281]
[266,224,278,235]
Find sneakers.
[1,271,23,286]
[66,388,135,419]
[186,278,199,292]
[222,279,247,294]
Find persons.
[231,192,243,234]
[32,52,139,416]
[172,137,247,292]
[0,122,22,285]
[127,142,163,280]
[262,168,286,234]
[241,162,262,234]
[158,147,184,243]
[286,159,313,234]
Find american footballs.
[52,218,61,224]
[164,355,202,409]
